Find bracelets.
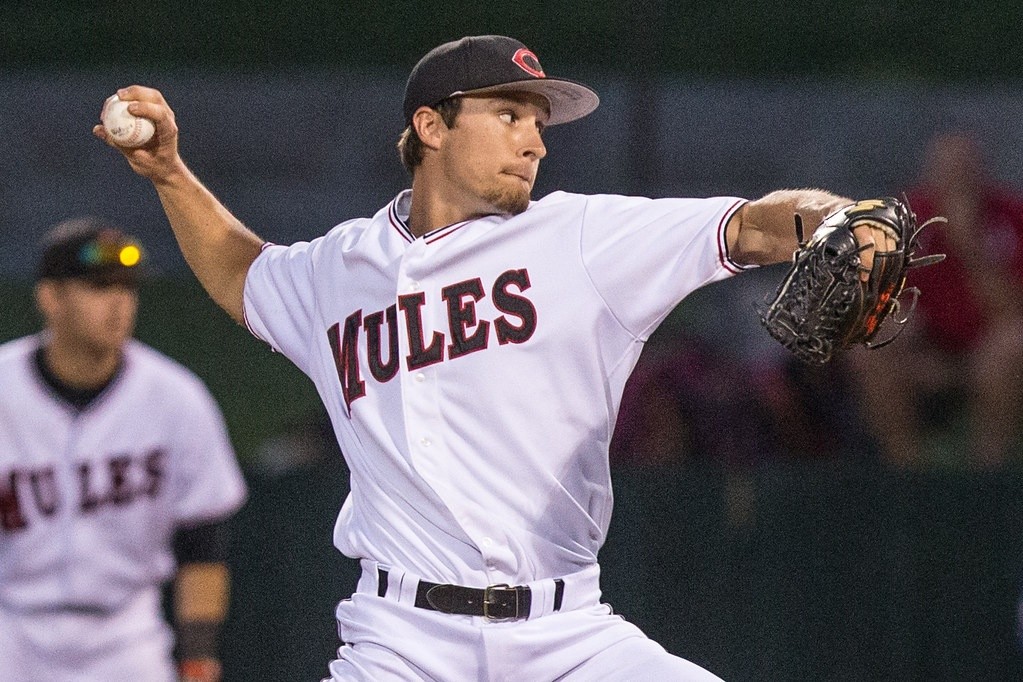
[176,619,231,664]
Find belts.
[377,569,565,623]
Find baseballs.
[100,94,156,149]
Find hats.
[401,35,600,125]
[37,214,156,284]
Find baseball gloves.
[760,197,917,373]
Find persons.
[1,217,249,681]
[92,35,946,682]
